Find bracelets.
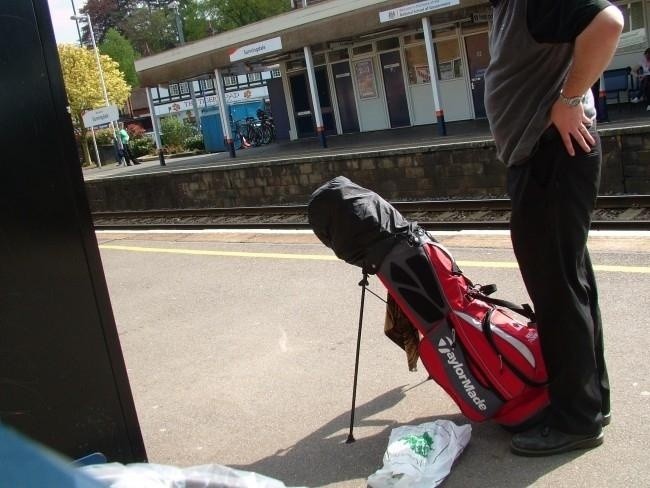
[559,89,584,107]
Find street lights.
[166,0,202,127]
[69,12,126,166]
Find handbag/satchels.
[306,173,555,432]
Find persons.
[630,48,650,112]
[484,0,626,457]
[118,122,141,166]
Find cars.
[142,130,164,144]
[178,122,203,143]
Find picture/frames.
[351,57,379,101]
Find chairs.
[603,65,635,112]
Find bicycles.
[221,107,276,149]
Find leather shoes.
[508,408,612,460]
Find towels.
[385,290,422,372]
